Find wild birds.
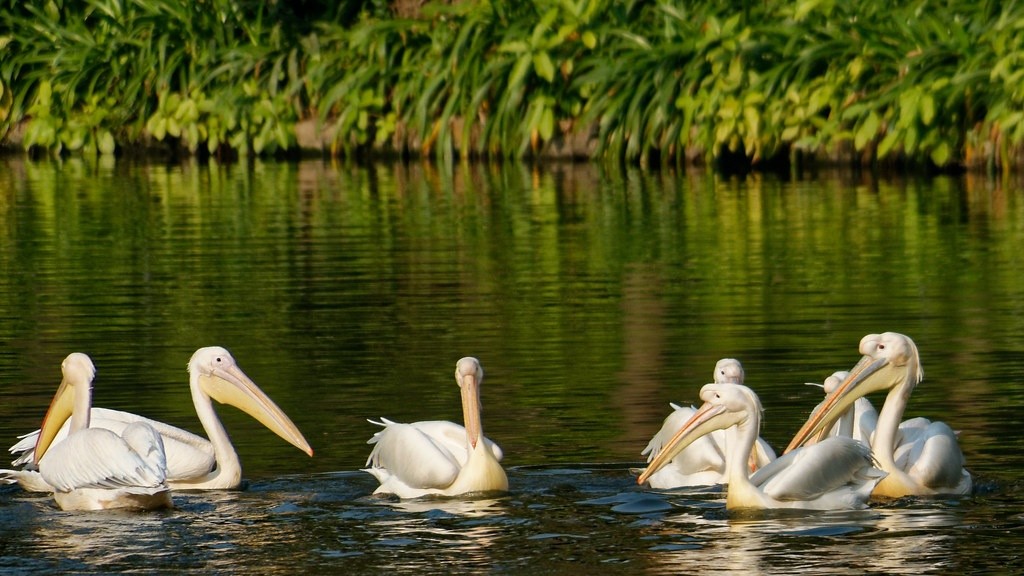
[637,331,972,511]
[359,356,509,503]
[0,345,312,511]
